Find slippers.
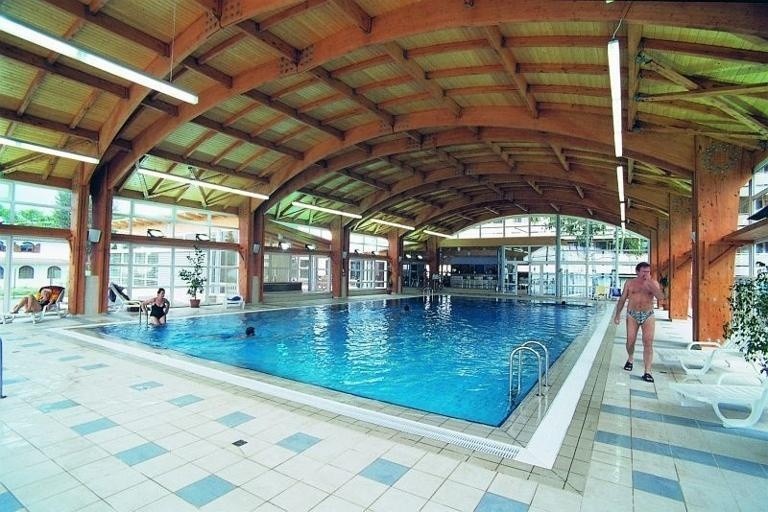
[642,373,654,382]
[624,362,632,371]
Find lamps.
[606,19,627,240]
[0,134,100,165]
[0,0,199,106]
[137,167,270,200]
[291,200,451,239]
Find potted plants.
[178,234,209,307]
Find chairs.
[669,368,768,429]
[656,334,757,375]
[0,286,65,325]
[223,295,245,310]
[108,282,144,311]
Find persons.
[9,289,52,313]
[200,327,255,341]
[141,288,170,326]
[403,303,411,311]
[614,262,663,383]
[422,270,428,289]
